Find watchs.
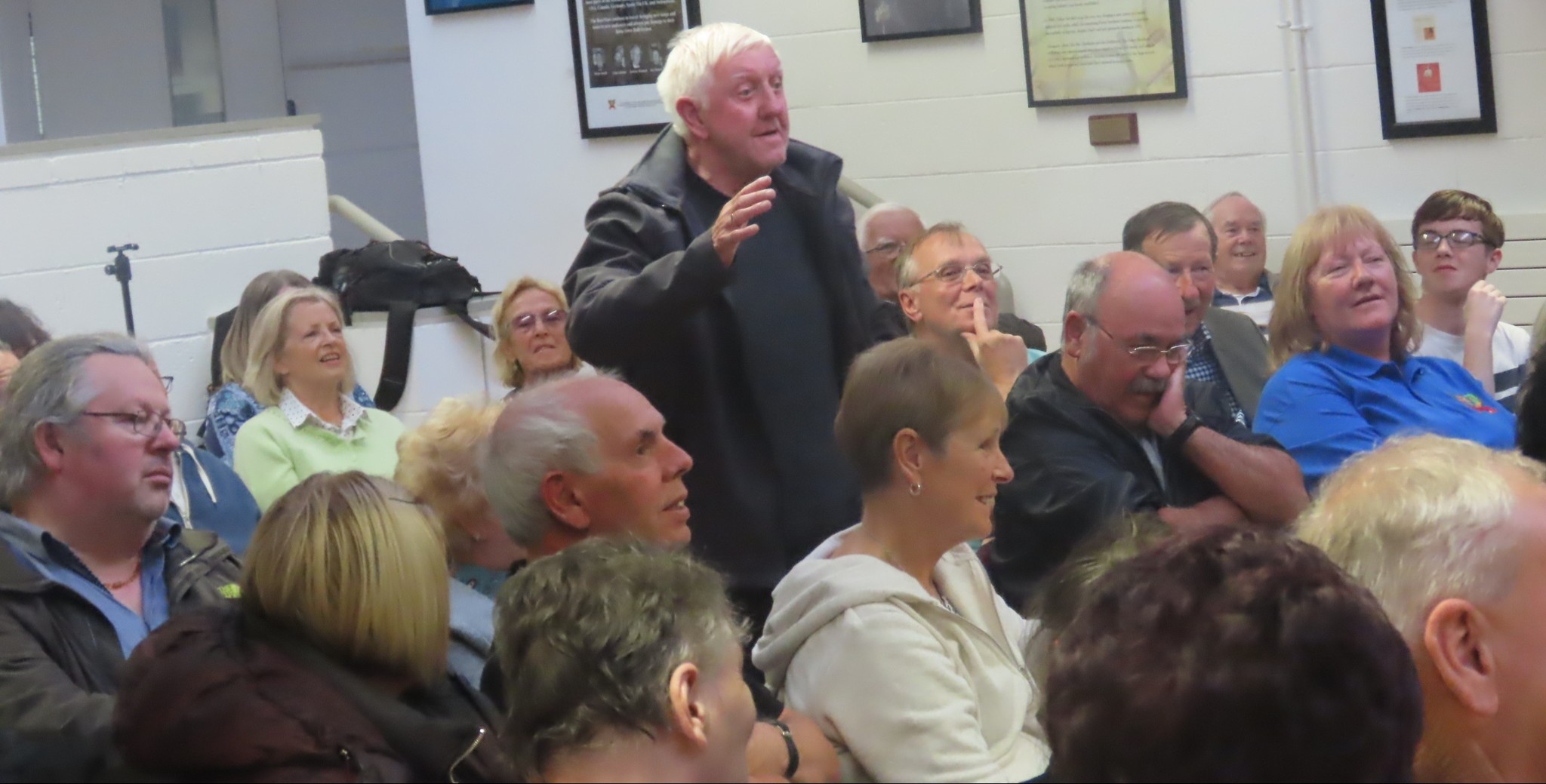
[1163,409,1204,463]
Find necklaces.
[98,560,143,591]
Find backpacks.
[313,237,496,411]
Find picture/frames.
[858,0,983,43]
[567,0,701,138]
[1370,0,1498,139]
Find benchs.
[1390,212,1546,336]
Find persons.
[1123,202,1272,426]
[561,22,889,719]
[0,185,1546,784]
[1252,205,1518,497]
[983,250,1309,623]
[229,284,408,516]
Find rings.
[731,213,741,226]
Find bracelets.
[771,721,799,778]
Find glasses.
[81,408,188,446]
[505,308,570,331]
[158,376,174,394]
[1414,230,1496,250]
[866,238,907,261]
[1081,313,1196,365]
[909,261,1002,288]
